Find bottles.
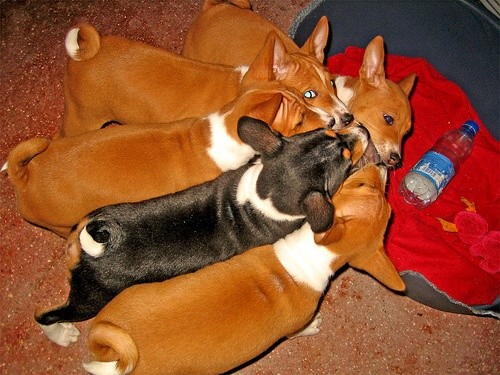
[399,120,479,209]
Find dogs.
[51,16,354,143]
[182,0,418,170]
[81,161,406,375]
[34,115,372,349]
[0,34,336,240]
